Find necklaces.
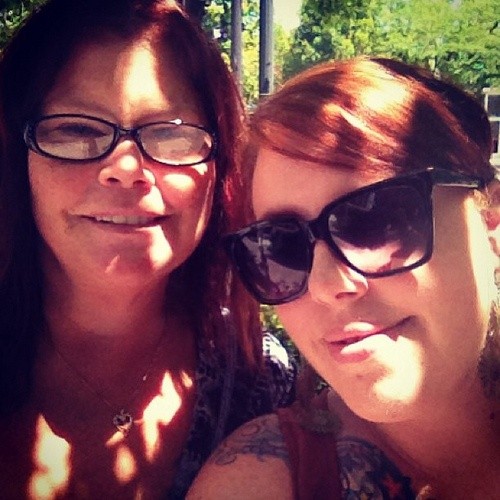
[107,398,138,436]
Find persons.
[185,56,498,498]
[0,0,309,497]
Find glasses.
[218,167,486,306]
[22,113,220,167]
